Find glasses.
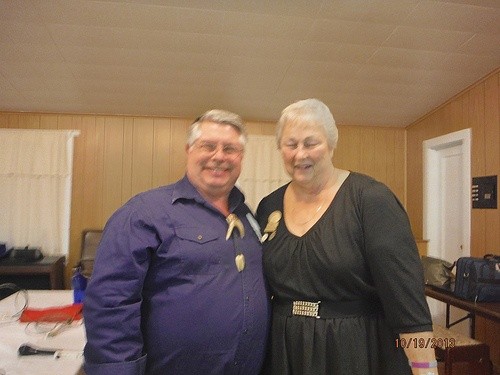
[193,143,243,154]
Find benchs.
[431,322,494,375]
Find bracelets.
[409,360,439,370]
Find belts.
[271,296,380,319]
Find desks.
[0,289,89,375]
[423,283,500,375]
[0,255,66,290]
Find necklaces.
[286,167,340,227]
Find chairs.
[72,228,105,279]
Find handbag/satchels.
[454,254,500,302]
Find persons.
[255,99,439,375]
[82,109,271,375]
